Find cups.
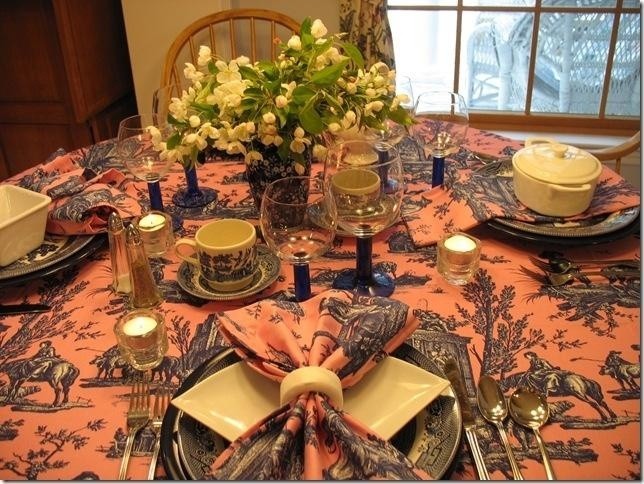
[173,218,258,292]
[130,211,173,256]
[330,169,380,219]
[113,309,169,370]
[511,138,602,217]
[437,231,481,286]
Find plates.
[178,244,282,301]
[472,160,640,245]
[0,183,52,267]
[2,236,109,287]
[170,359,452,446]
[159,345,464,481]
[308,193,403,237]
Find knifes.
[443,359,490,481]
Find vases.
[244,134,312,229]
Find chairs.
[155,6,302,131]
[468,0,640,116]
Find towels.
[13,151,150,236]
[206,287,438,481]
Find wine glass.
[118,114,183,233]
[366,75,414,192]
[325,139,406,299]
[412,90,470,188]
[259,175,339,300]
[152,83,217,210]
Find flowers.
[147,19,411,174]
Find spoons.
[474,373,523,479]
[509,387,556,480]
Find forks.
[146,384,176,480]
[528,254,638,272]
[518,264,640,287]
[117,376,151,481]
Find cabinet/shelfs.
[0,0,138,180]
